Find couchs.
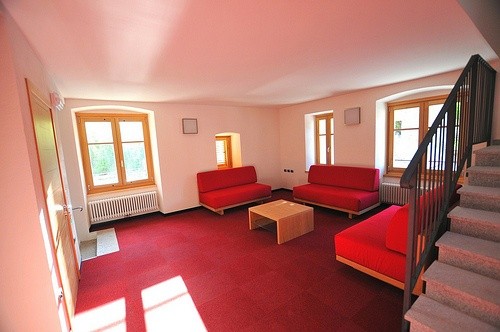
[293,165,381,218]
[196,165,271,216]
[334,183,463,297]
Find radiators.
[381,183,433,206]
[87,191,160,225]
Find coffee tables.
[248,199,313,244]
